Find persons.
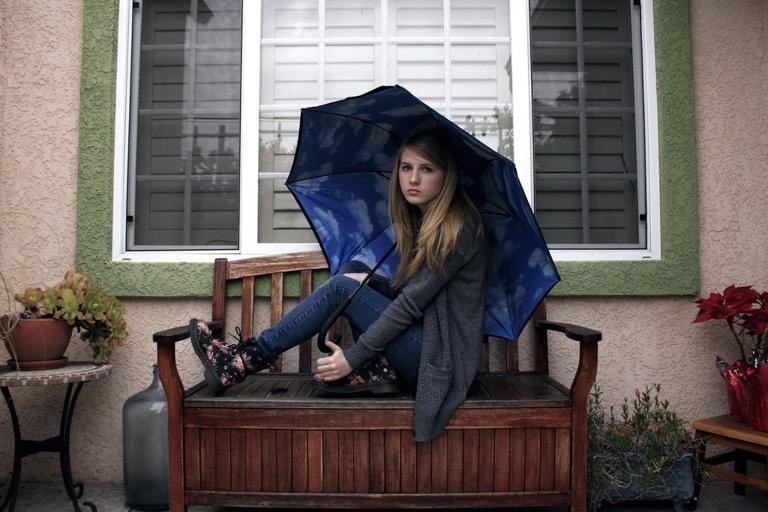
[190,128,491,443]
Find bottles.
[123,363,170,512]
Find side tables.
[0,362,113,511]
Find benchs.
[152,252,603,511]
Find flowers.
[692,284,768,364]
[587,380,717,502]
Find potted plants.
[0,270,129,368]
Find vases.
[590,436,697,512]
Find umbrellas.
[284,84,562,344]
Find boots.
[319,354,397,394]
[190,318,272,396]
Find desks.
[684,414,767,512]
